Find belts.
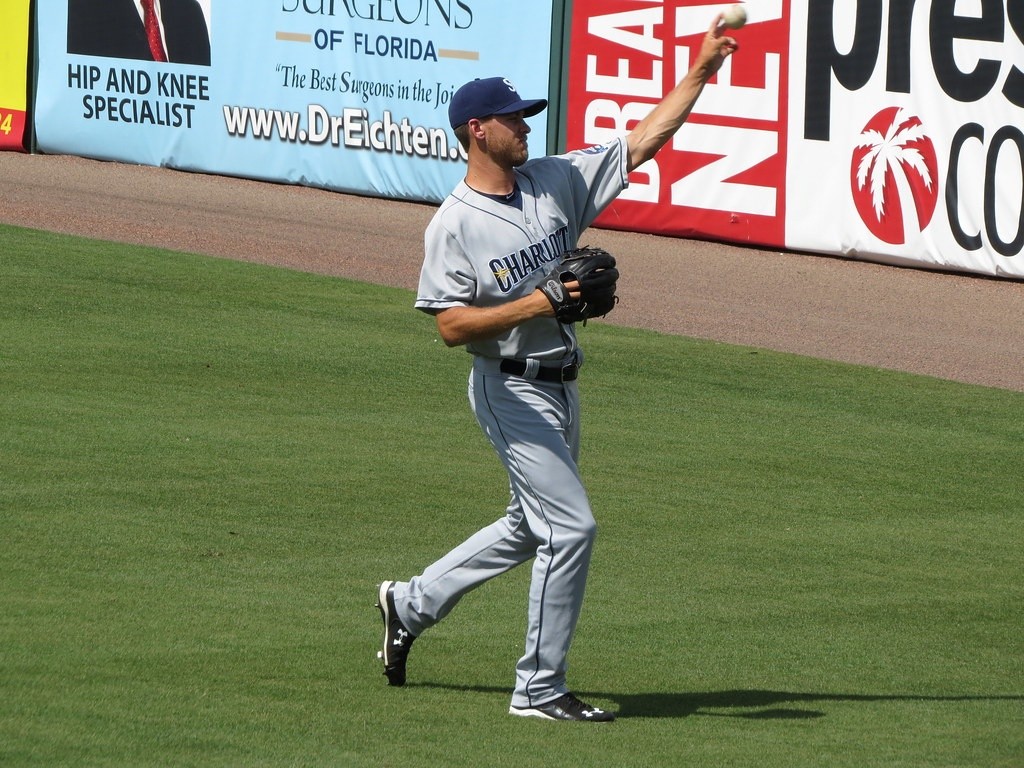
[500,358,579,384]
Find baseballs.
[723,6,747,28]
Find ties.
[142,0,167,62]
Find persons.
[67,0,211,66]
[380,12,737,723]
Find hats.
[448,77,548,130]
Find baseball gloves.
[539,242,620,329]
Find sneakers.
[374,580,416,687]
[508,692,615,722]
